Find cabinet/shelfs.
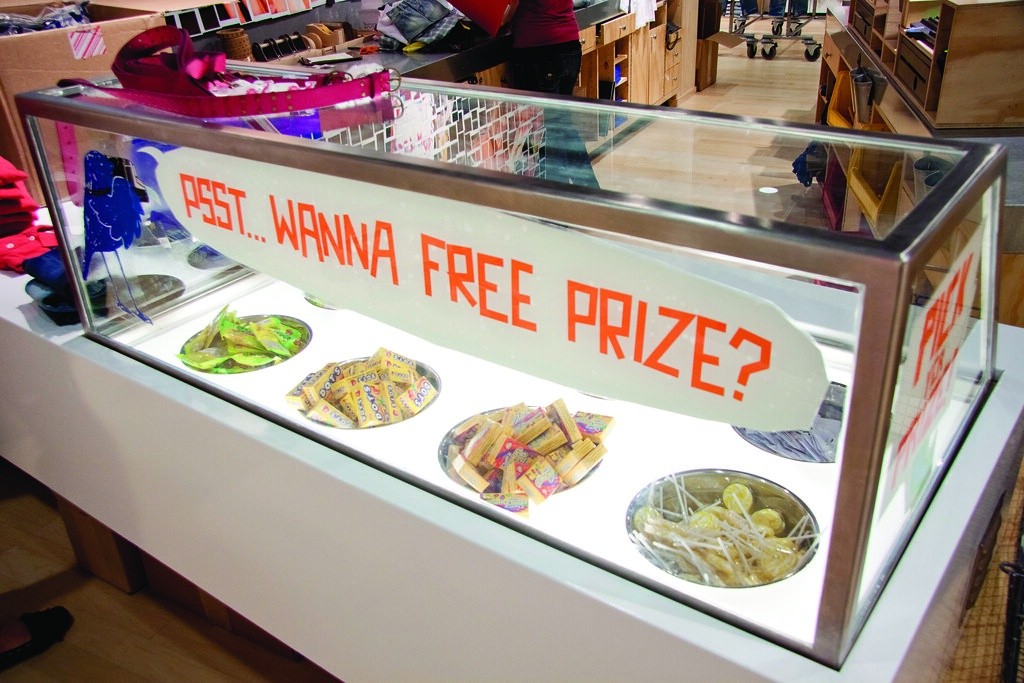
[461,0,698,164]
[815,0,1024,329]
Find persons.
[502,0,600,193]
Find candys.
[177,304,305,373]
[633,484,805,584]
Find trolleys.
[730,1,827,60]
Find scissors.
[348,46,379,55]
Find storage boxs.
[822,158,847,230]
[827,70,866,148]
[695,33,746,91]
[448,399,615,520]
[846,124,905,226]
[0,0,229,205]
[286,349,438,428]
